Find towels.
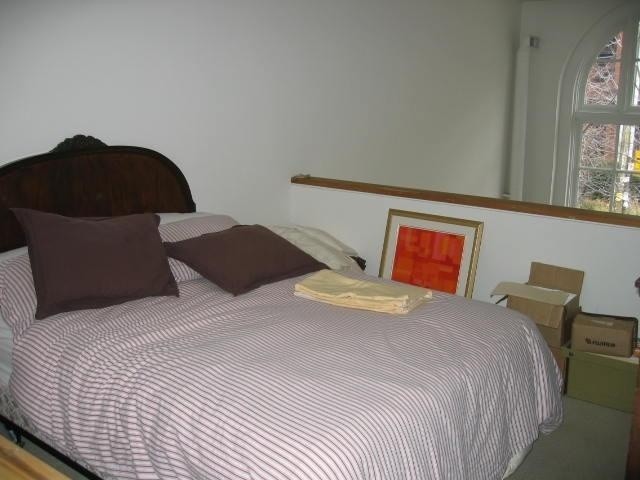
[295,269,435,315]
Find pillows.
[164,224,331,297]
[9,206,180,320]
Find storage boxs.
[560,340,638,413]
[547,347,566,394]
[490,261,585,348]
[571,311,638,356]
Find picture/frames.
[378,208,484,299]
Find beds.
[0,135,563,480]
